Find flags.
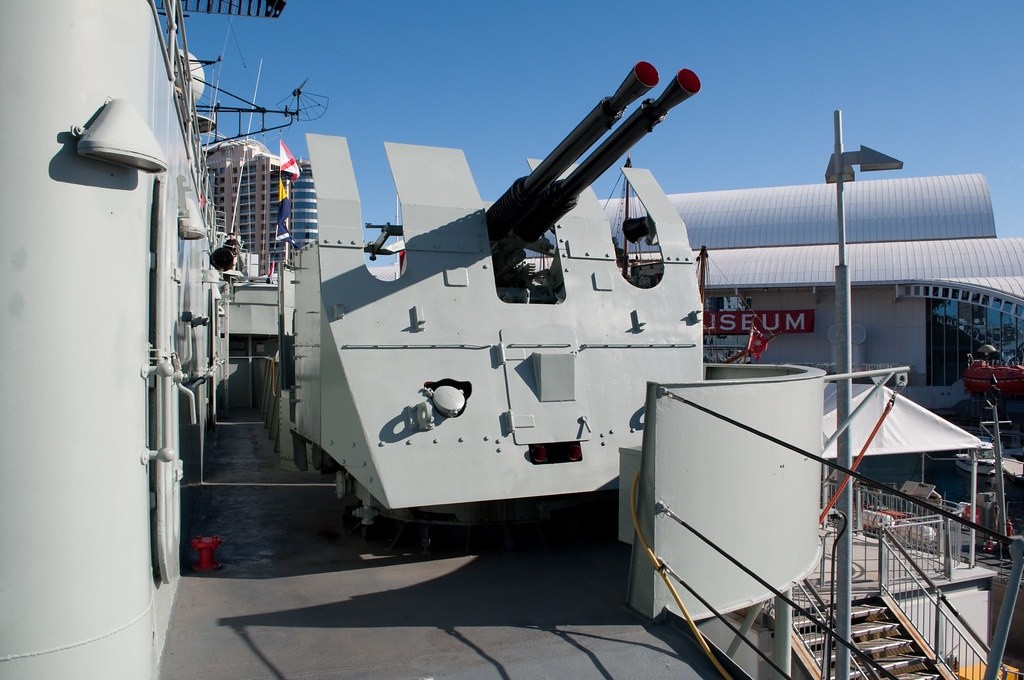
[747,325,769,361]
[277,178,299,249]
[280,140,300,180]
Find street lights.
[822,148,904,680]
